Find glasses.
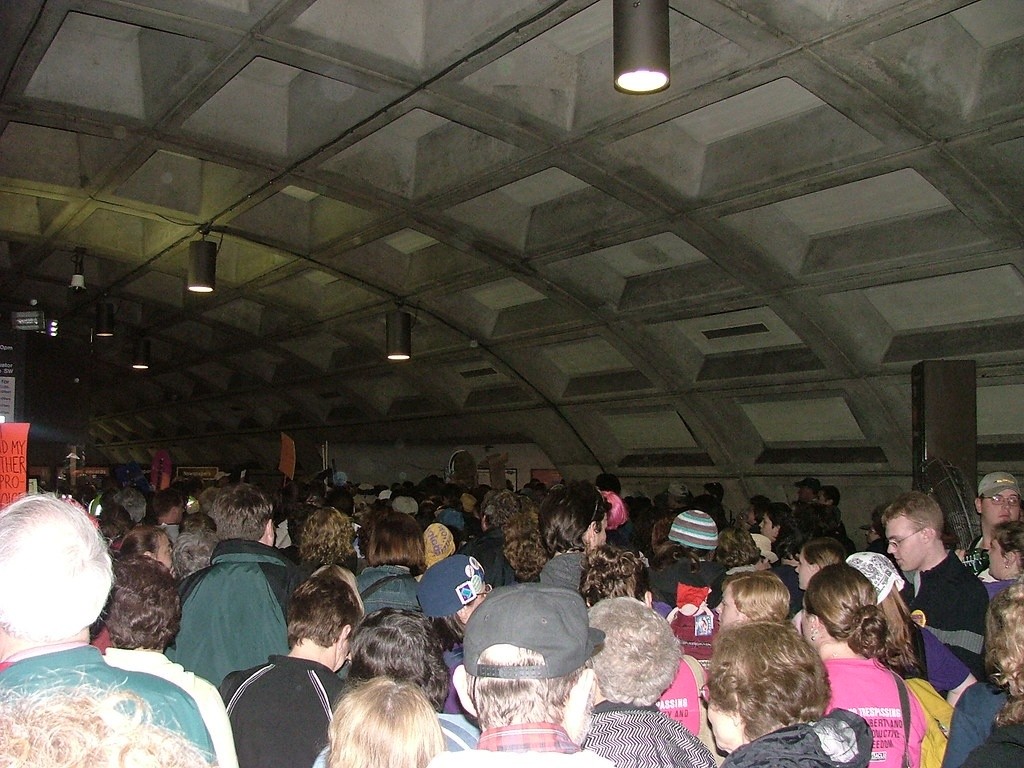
[986,494,1023,506]
[547,484,600,522]
[701,682,711,693]
[887,528,925,547]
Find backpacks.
[655,655,705,741]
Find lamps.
[187,223,216,293]
[67,246,89,292]
[96,302,114,336]
[385,296,411,360]
[611,0,671,96]
[132,340,150,370]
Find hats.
[704,483,724,502]
[978,472,1021,498]
[463,583,606,679]
[796,478,820,490]
[750,534,778,564]
[859,522,876,532]
[391,495,419,515]
[668,510,718,550]
[379,490,391,500]
[423,523,454,568]
[667,481,689,497]
[460,494,477,513]
[418,554,492,617]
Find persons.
[847,552,977,709]
[708,621,872,768]
[0,465,855,768]
[801,562,927,768]
[957,584,1024,768]
[982,520,1024,601]
[882,492,990,682]
[866,522,907,581]
[957,471,1024,577]
